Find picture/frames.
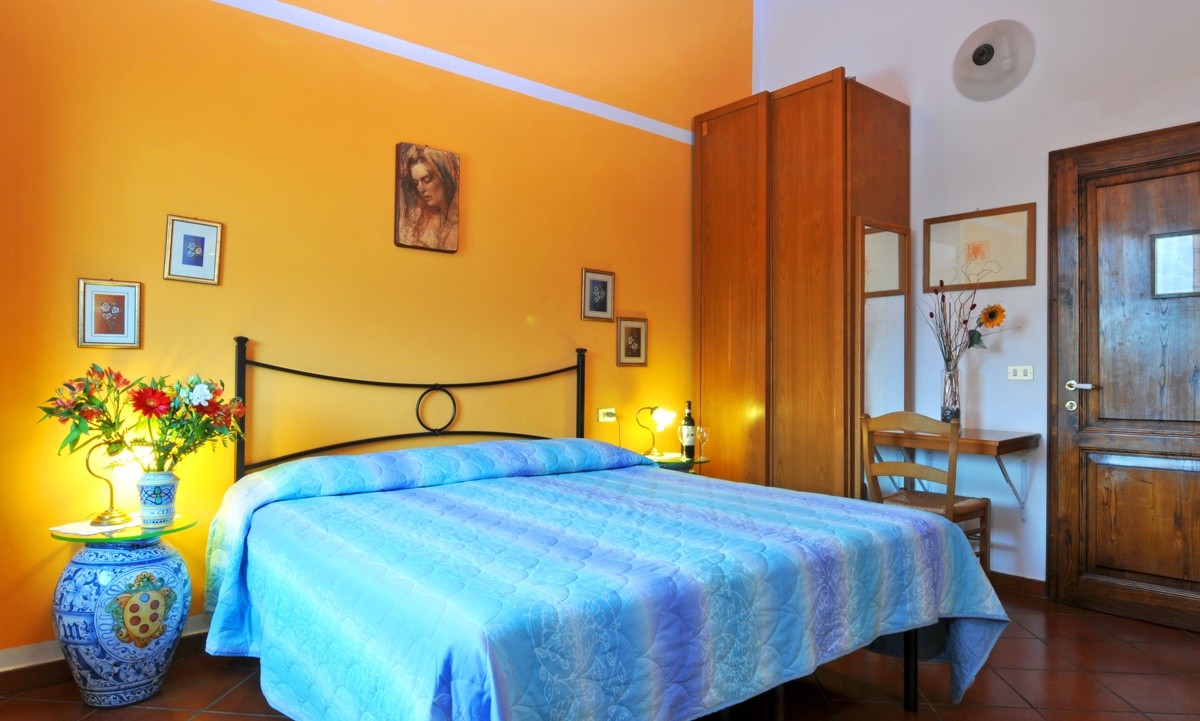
[77,280,142,350]
[164,214,224,286]
[616,316,649,367]
[923,201,1037,295]
[581,269,615,322]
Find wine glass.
[696,426,710,460]
[678,425,692,461]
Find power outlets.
[598,408,616,423]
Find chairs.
[860,413,991,583]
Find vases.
[50,539,190,708]
[940,371,960,424]
[139,473,178,526]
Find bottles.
[681,401,695,458]
[51,537,193,708]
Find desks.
[871,430,1042,506]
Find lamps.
[637,405,677,457]
[85,435,152,527]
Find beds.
[201,336,1010,721]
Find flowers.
[40,366,247,470]
[922,259,1006,420]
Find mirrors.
[854,218,914,494]
